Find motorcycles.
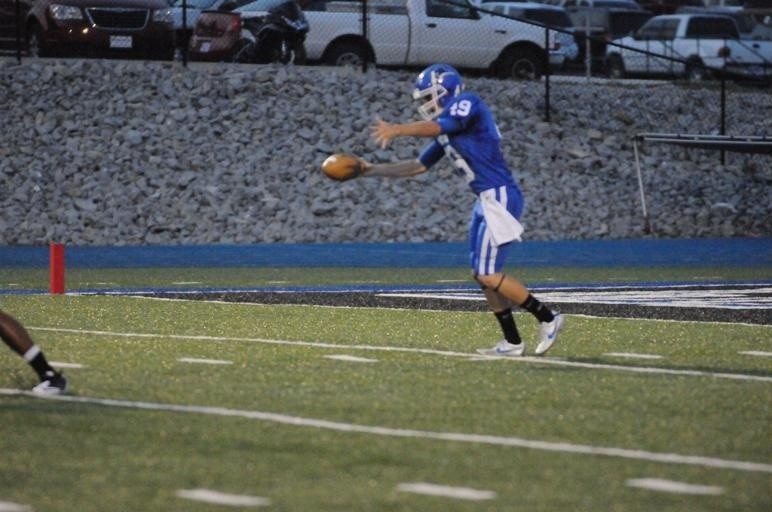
[226,2,310,67]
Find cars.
[562,7,653,73]
[539,0,642,10]
[172,0,290,62]
[543,0,651,15]
[0,0,175,60]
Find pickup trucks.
[301,1,566,82]
[606,15,772,81]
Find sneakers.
[475,338,527,358]
[31,375,68,397]
[534,308,565,356]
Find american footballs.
[320,155,361,181]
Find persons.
[355,63,563,356]
[0,312,66,394]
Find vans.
[479,3,580,64]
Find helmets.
[411,62,466,123]
